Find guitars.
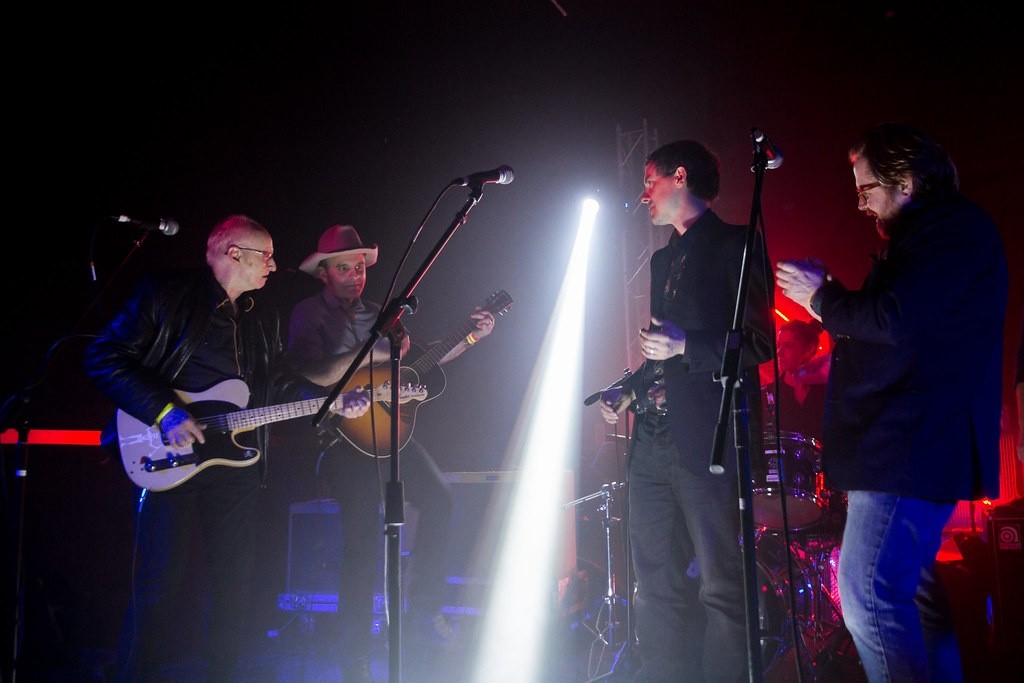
[326,288,514,462]
[114,376,429,494]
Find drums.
[746,432,834,675]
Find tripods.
[559,479,638,683]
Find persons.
[599,136,777,683]
[759,318,830,445]
[82,215,371,683]
[776,120,1009,683]
[287,224,454,615]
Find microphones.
[452,165,516,186]
[754,129,784,169]
[112,215,180,235]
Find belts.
[647,404,667,417]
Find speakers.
[286,499,419,597]
[983,516,1024,683]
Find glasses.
[224,247,273,263]
[857,181,881,205]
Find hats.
[301,224,379,280]
